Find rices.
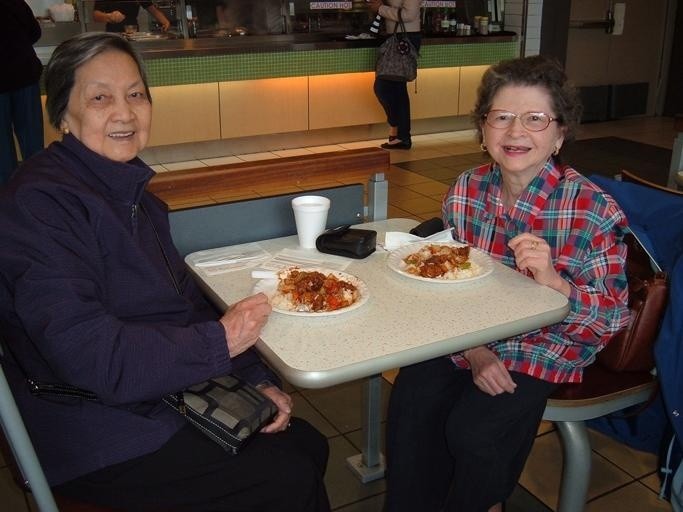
[267,271,358,313]
[403,245,483,280]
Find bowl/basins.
[47,7,75,22]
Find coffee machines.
[281,0,383,35]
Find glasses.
[484,109,559,132]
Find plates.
[252,268,371,317]
[386,241,496,283]
[40,19,50,23]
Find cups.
[125,25,137,34]
[291,195,331,249]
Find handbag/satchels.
[375,33,417,82]
[163,372,279,457]
[597,234,668,375]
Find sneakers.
[381,139,412,150]
[389,136,397,141]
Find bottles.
[472,15,489,36]
[185,5,197,39]
[433,11,457,35]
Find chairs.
[1,327,105,509]
[542,174,683,512]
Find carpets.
[547,137,672,190]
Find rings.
[531,239,538,249]
[286,421,291,428]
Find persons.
[382,54,630,510]
[0,0,43,184]
[362,0,421,150]
[92,0,169,32]
[0,33,332,510]
